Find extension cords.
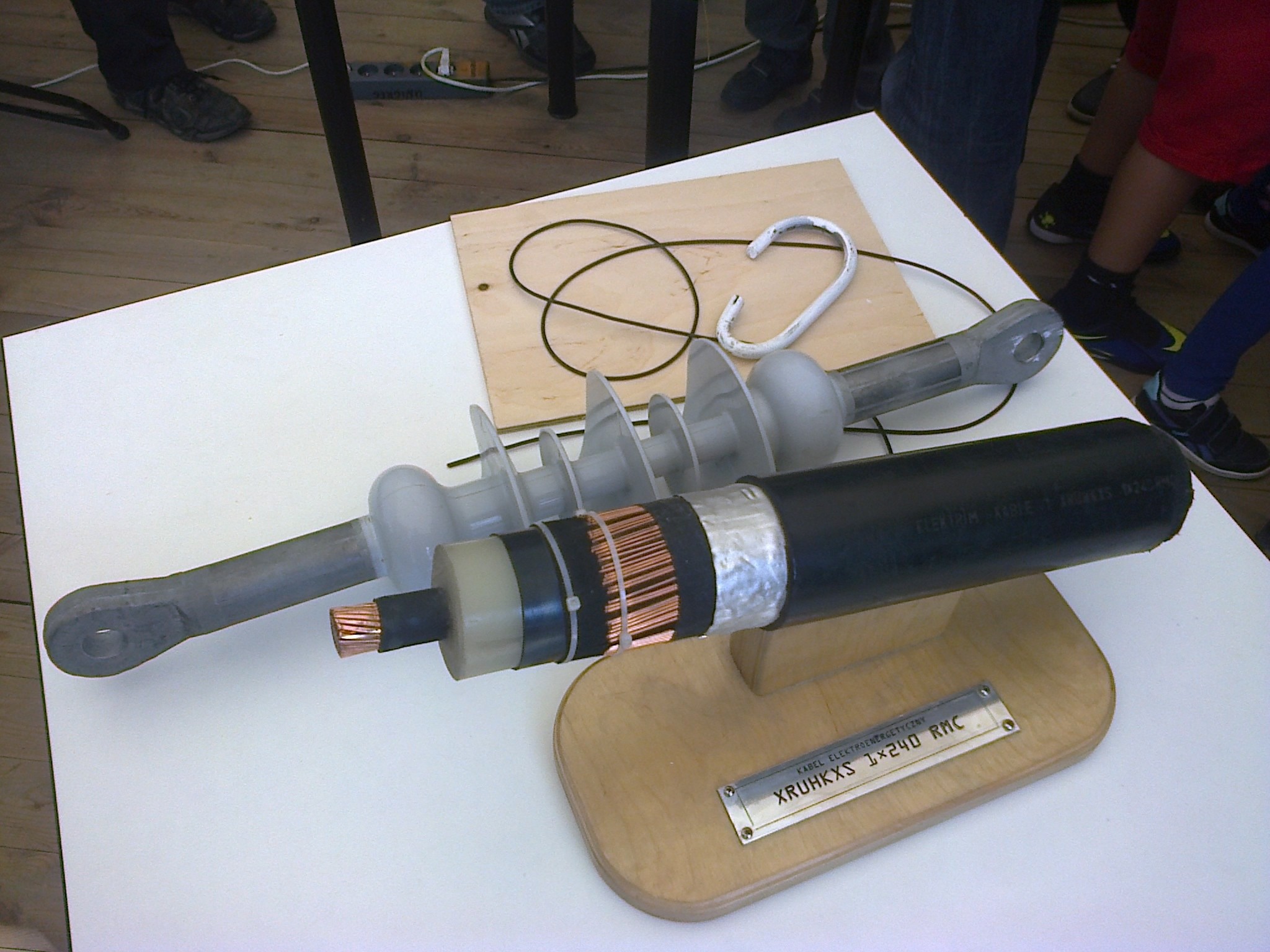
[345,61,492,99]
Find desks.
[2,108,1270,952]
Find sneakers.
[1129,369,1270,479]
[110,66,252,142]
[168,0,278,42]
[1028,180,1182,260]
[1052,288,1190,376]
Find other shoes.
[1204,192,1270,257]
[773,86,850,130]
[718,43,814,111]
[484,5,596,74]
[1067,66,1116,124]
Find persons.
[483,0,597,77]
[67,0,277,143]
[718,0,1269,480]
